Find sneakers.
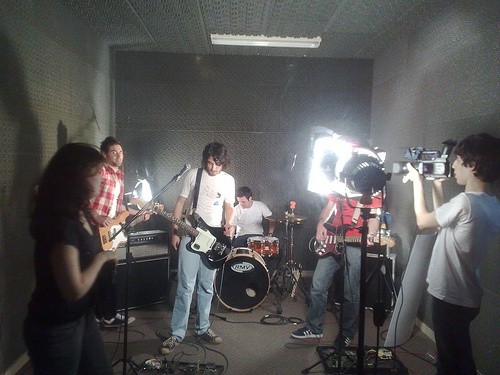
[160,336,179,354]
[291,327,323,338]
[96,313,136,327]
[333,333,353,346]
[194,327,222,344]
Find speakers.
[114,256,171,312]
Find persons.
[403,133,500,375]
[78,137,136,327]
[231,186,274,249]
[290,136,384,348]
[24,143,117,375]
[160,141,236,354]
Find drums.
[246,236,279,257]
[214,247,270,312]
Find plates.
[145,359,163,370]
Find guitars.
[314,222,397,257]
[97,202,160,252]
[130,197,231,263]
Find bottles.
[289,200,296,217]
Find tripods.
[269,224,311,303]
[109,177,178,375]
[301,199,361,375]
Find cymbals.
[266,215,308,222]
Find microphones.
[175,164,191,181]
